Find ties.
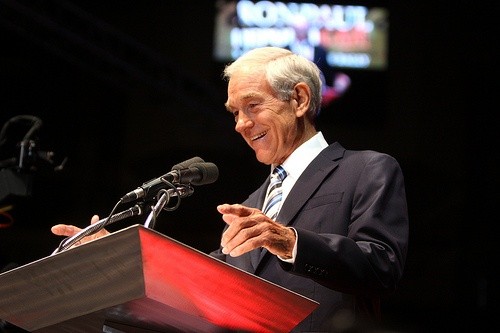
[261,165,287,222]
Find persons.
[50,47,409,333]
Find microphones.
[119,157,205,203]
[162,162,219,185]
